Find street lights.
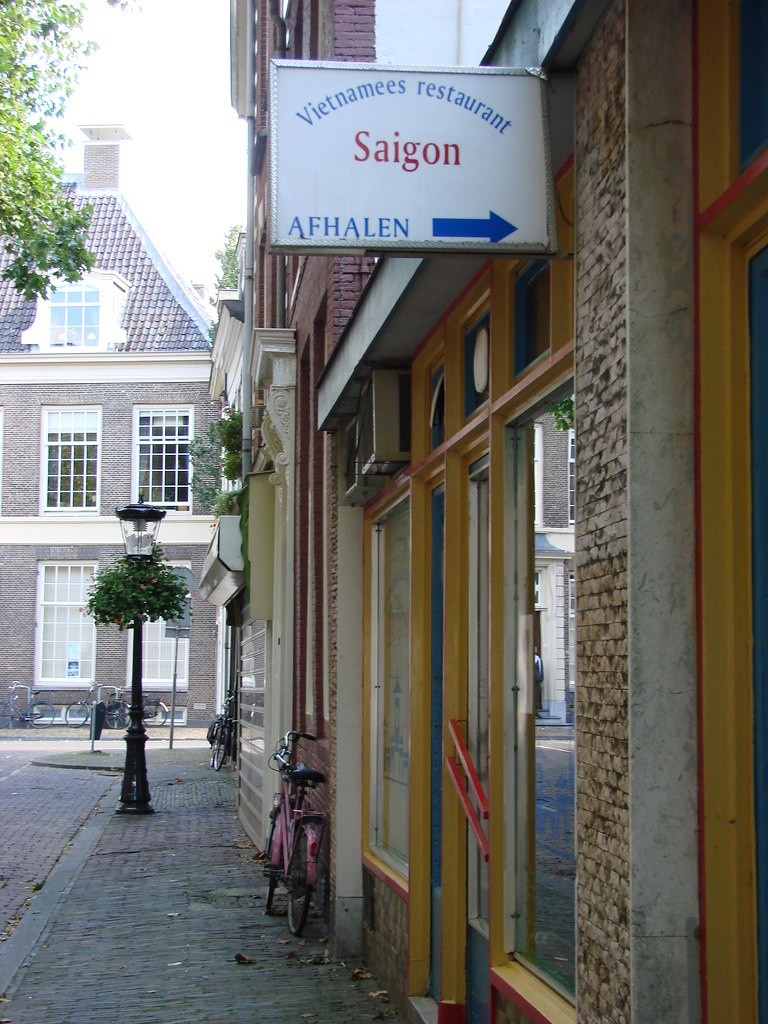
[113,487,168,815]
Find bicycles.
[65,681,168,731]
[204,689,239,771]
[0,679,57,729]
[258,732,330,938]
